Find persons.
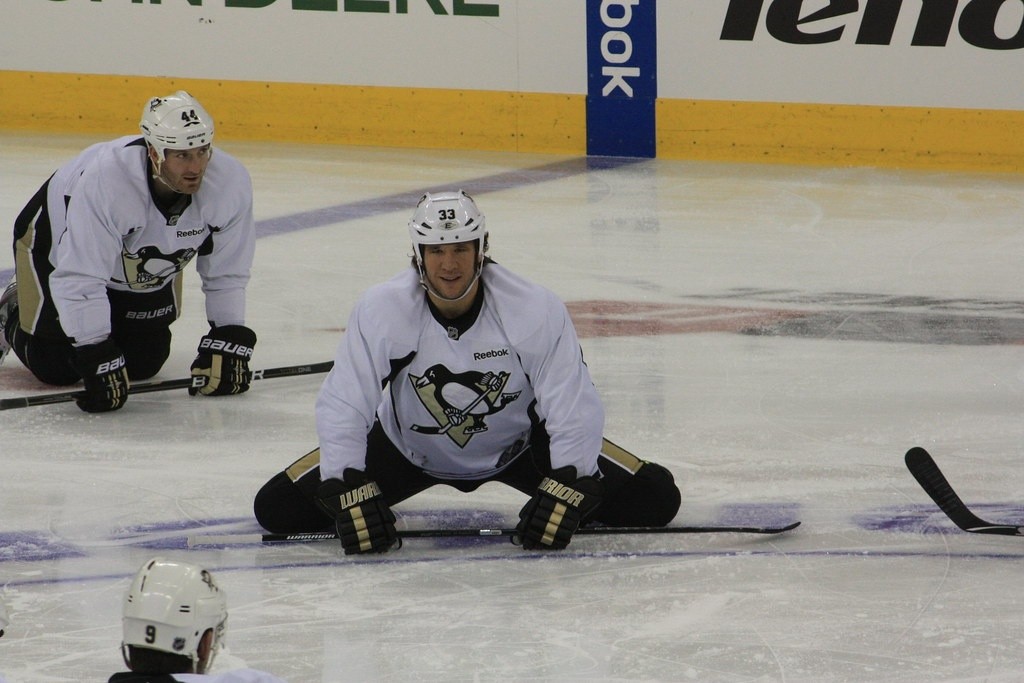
[0,87,260,414]
[107,557,283,682]
[252,190,682,556]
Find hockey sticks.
[902,444,1024,538]
[260,519,802,543]
[0,359,335,413]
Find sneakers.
[0,282,19,366]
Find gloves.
[313,468,402,556]
[188,325,257,396]
[68,333,130,413]
[510,465,604,550]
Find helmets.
[139,90,214,163]
[122,557,228,671]
[407,189,485,266]
